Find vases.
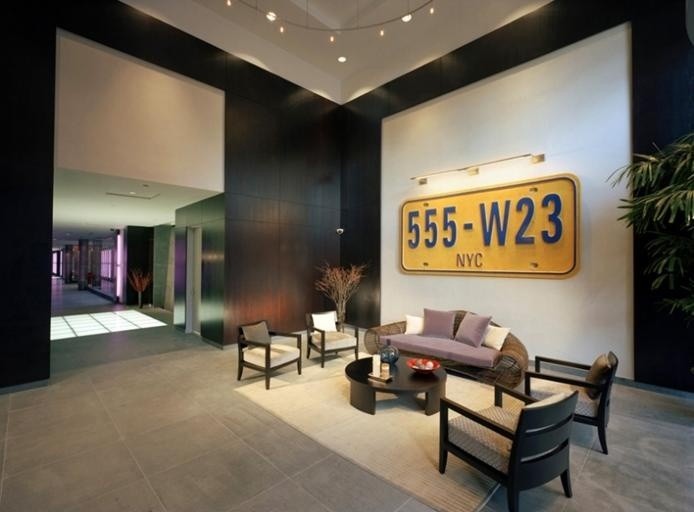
[138,291,142,309]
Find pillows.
[453,312,491,345]
[485,322,510,350]
[404,314,425,334]
[422,307,454,338]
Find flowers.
[128,270,153,292]
[316,265,361,327]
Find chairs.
[237,319,303,390]
[440,385,580,511]
[493,349,618,456]
[306,313,360,370]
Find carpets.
[233,351,573,511]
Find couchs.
[363,307,528,391]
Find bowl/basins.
[406,358,440,375]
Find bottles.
[380,363,390,379]
[372,354,380,378]
[377,339,401,366]
